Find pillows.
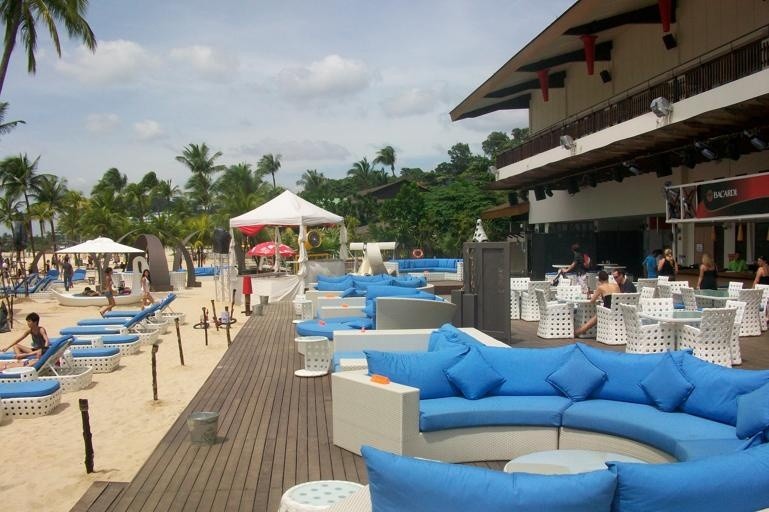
[641,350,693,409]
[736,379,769,439]
[446,344,506,400]
[546,343,608,403]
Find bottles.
[586,286,591,299]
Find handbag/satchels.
[660,257,674,276]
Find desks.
[284,477,370,511]
[503,450,649,477]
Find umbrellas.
[53,236,145,253]
[246,242,296,269]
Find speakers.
[213,228,232,254]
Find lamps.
[559,135,576,150]
[650,97,673,118]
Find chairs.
[0,287,184,420]
[108,266,224,287]
[3,269,85,294]
[512,259,769,370]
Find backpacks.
[580,252,594,270]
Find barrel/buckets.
[252,304,263,316]
[186,411,219,446]
[260,295,268,304]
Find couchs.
[393,260,463,291]
[344,350,769,512]
[291,261,466,371]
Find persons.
[0,312,49,359]
[0,253,131,297]
[560,243,678,337]
[139,269,155,312]
[99,268,115,319]
[695,254,719,292]
[727,252,749,273]
[751,254,769,290]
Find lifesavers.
[412,249,423,259]
